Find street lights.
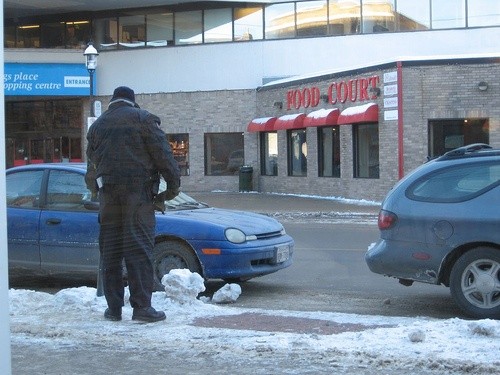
[83,44,99,117]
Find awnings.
[302,108,339,128]
[273,113,305,130]
[245,116,276,131]
[336,103,379,126]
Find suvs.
[364,142,500,319]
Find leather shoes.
[104,306,122,321]
[132,307,166,321]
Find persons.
[84,86,182,323]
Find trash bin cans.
[237,165,254,193]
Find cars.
[5,160,296,294]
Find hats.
[110,86,135,101]
